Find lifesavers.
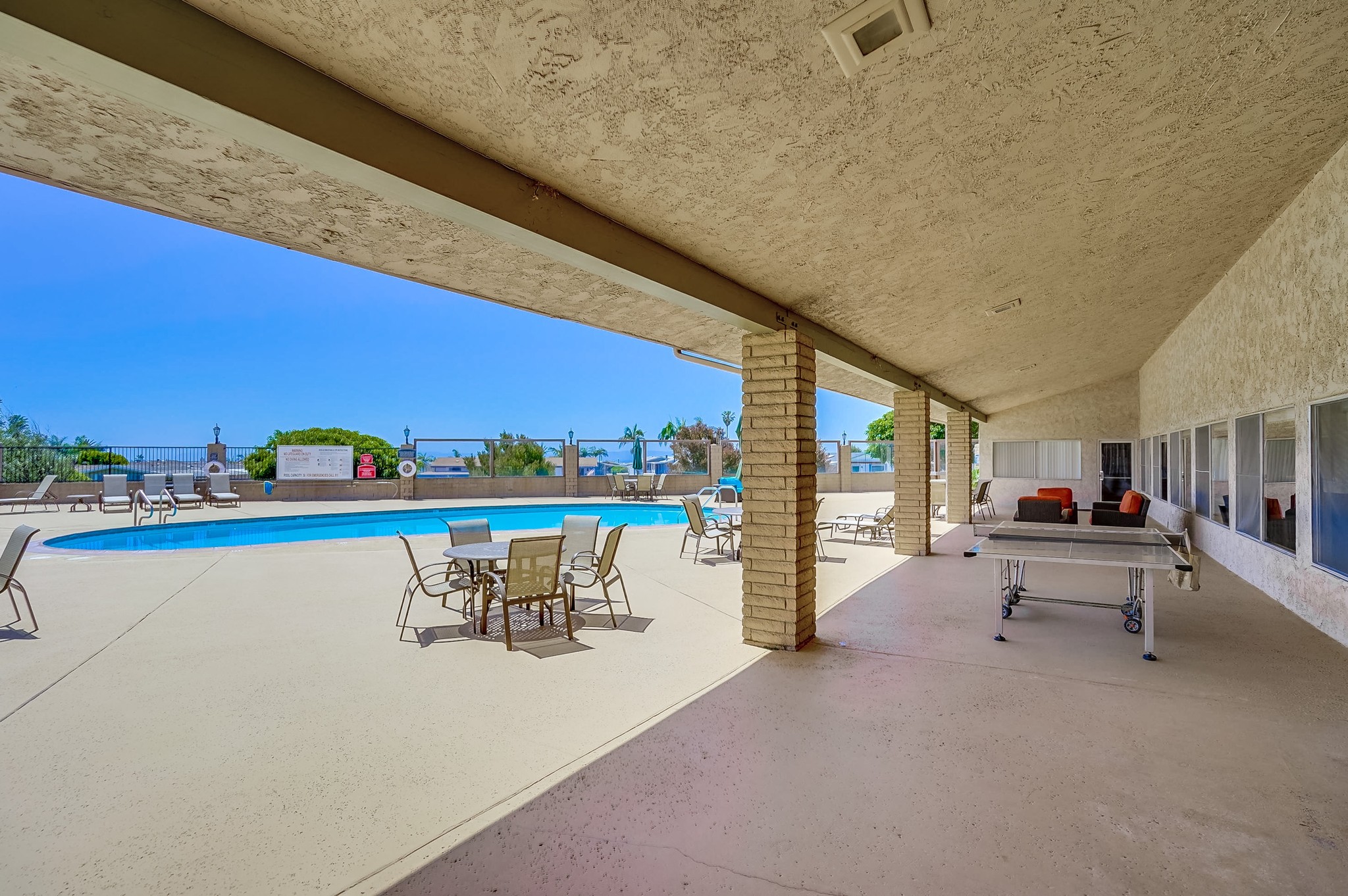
[398,460,417,477]
[204,460,225,479]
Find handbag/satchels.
[1166,531,1201,591]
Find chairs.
[932,501,946,519]
[99,474,133,512]
[678,493,743,564]
[814,496,895,562]
[604,471,669,502]
[140,474,171,509]
[1013,487,1078,524]
[0,474,60,514]
[972,479,995,521]
[0,525,43,630]
[1090,490,1151,528]
[170,473,203,510]
[1265,493,1295,551]
[391,514,632,651]
[206,472,241,508]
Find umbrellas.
[632,436,643,479]
[735,413,743,481]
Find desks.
[713,507,742,561]
[623,479,654,500]
[961,518,1194,663]
[442,541,567,634]
[66,494,95,512]
